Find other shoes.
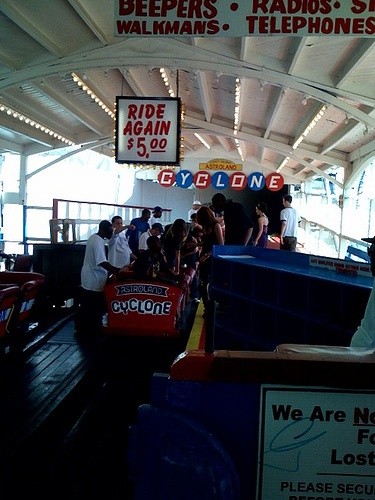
[192,297,201,303]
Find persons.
[126,200,225,318]
[280,196,301,252]
[351,236,375,348]
[73,216,137,334]
[212,193,255,246]
[254,202,269,248]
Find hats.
[215,210,224,215]
[152,206,163,212]
[152,223,165,232]
[361,236,374,243]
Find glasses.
[367,246,374,256]
